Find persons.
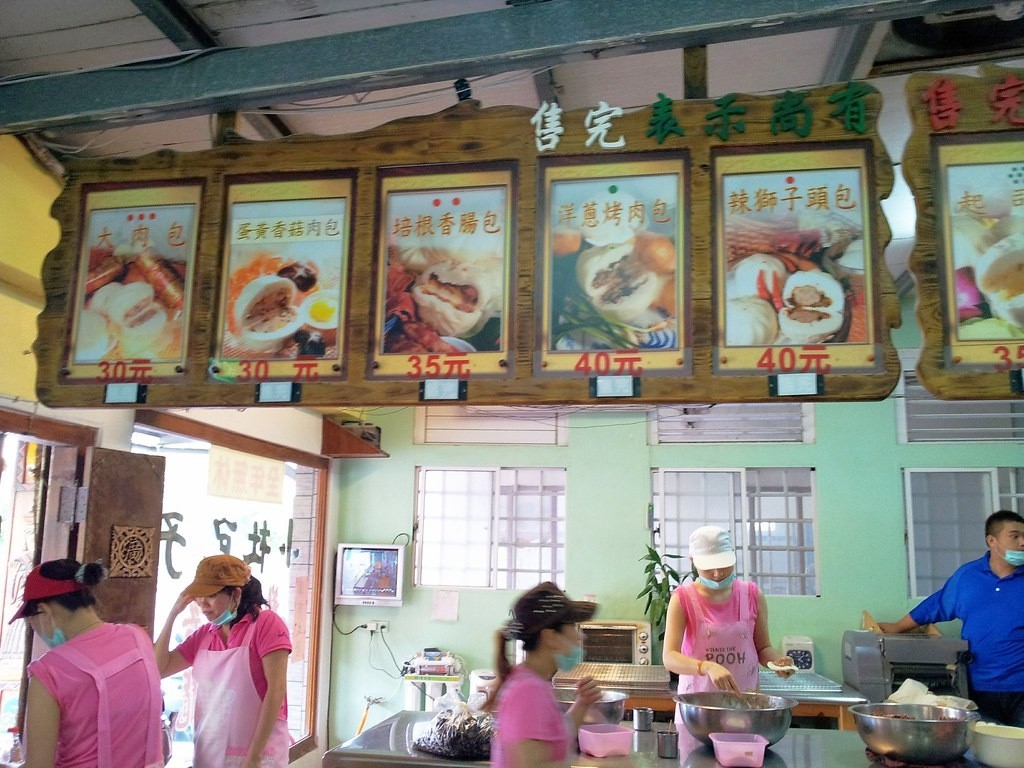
[5,557,166,768]
[479,581,603,768]
[876,510,1024,728]
[661,524,796,724]
[156,553,294,768]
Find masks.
[986,540,1024,569]
[37,611,65,650]
[547,631,586,671]
[698,570,738,590]
[209,591,242,627]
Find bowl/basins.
[708,732,770,768]
[577,723,635,759]
[847,702,981,760]
[550,687,630,727]
[672,690,799,747]
[972,724,1024,768]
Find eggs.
[306,297,339,329]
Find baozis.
[719,251,845,344]
[402,245,501,338]
[234,275,305,343]
[573,190,662,324]
[951,210,1024,340]
[74,282,168,351]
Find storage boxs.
[703,730,770,767]
[577,723,635,757]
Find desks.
[623,670,866,730]
[324,707,983,768]
[402,672,466,712]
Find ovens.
[576,619,653,667]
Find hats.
[7,563,87,626]
[688,524,737,572]
[510,580,599,638]
[180,553,252,598]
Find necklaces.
[70,620,101,638]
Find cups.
[656,731,678,758]
[632,707,654,731]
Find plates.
[301,290,338,330]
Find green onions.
[551,291,671,351]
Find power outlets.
[369,620,389,633]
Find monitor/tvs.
[335,543,404,606]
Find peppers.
[756,233,822,311]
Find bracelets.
[696,661,706,676]
